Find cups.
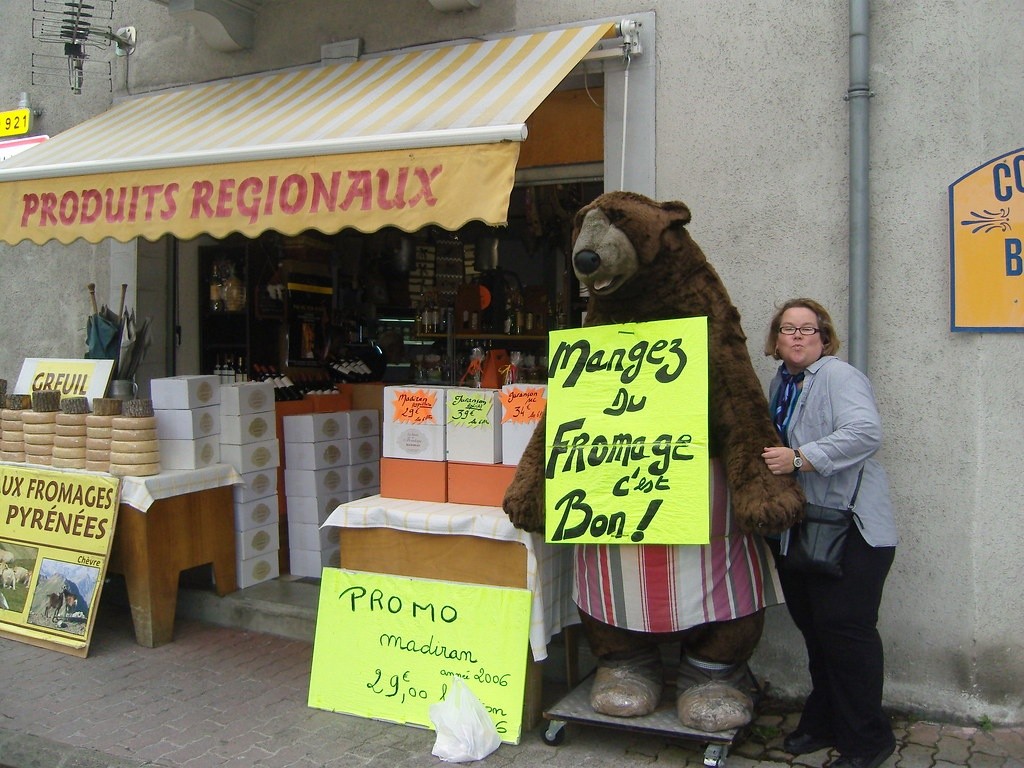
[110,380,139,401]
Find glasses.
[778,326,822,336]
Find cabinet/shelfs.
[195,246,550,399]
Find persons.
[761,298,899,768]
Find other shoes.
[828,741,897,768]
[783,729,837,755]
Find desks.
[317,492,580,733]
[109,461,236,647]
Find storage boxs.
[151,375,547,589]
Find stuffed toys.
[504,191,808,732]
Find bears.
[500,189,808,736]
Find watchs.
[794,449,803,471]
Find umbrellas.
[85,283,151,380]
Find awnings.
[1,24,619,248]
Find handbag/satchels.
[775,502,864,580]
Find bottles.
[546,299,568,336]
[463,309,478,330]
[222,353,228,383]
[416,303,455,333]
[236,356,248,382]
[224,262,243,311]
[287,367,339,394]
[326,356,376,383]
[458,339,494,382]
[210,260,223,312]
[417,364,442,384]
[252,360,303,401]
[255,238,286,315]
[229,352,236,383]
[214,352,222,384]
[504,298,537,336]
